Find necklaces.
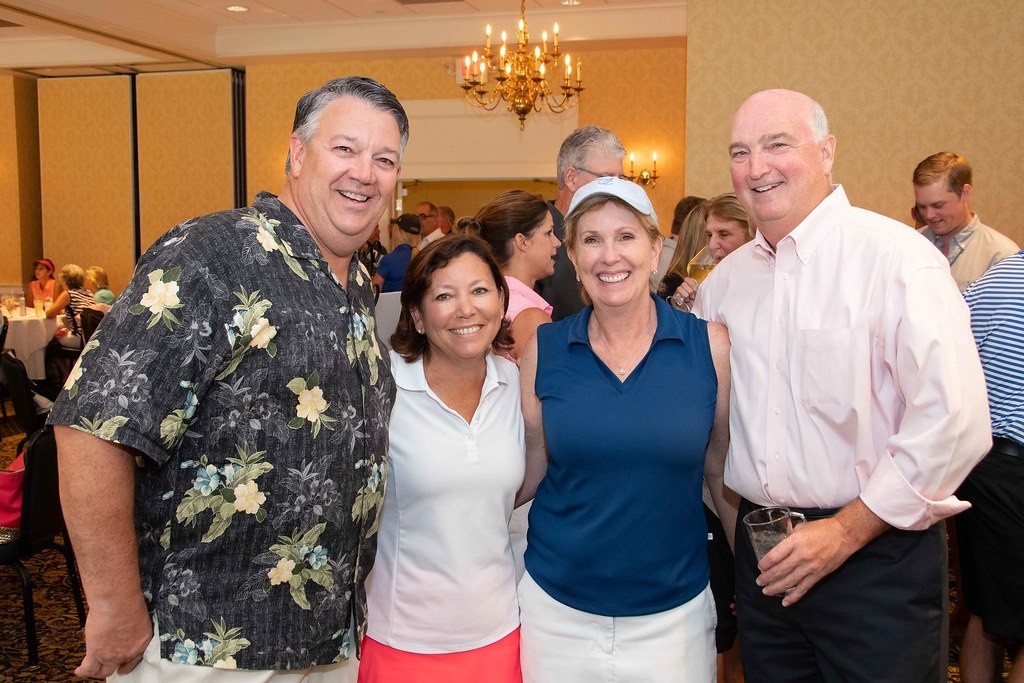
[602,335,632,374]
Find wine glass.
[666,244,721,312]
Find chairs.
[0,302,109,663]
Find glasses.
[573,166,630,181]
[417,213,433,221]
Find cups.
[45,297,53,312]
[743,506,794,575]
[33,295,44,317]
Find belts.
[990,435,1024,457]
[737,496,829,526]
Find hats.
[562,176,659,234]
[389,213,421,235]
[33,259,54,275]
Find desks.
[2,306,62,380]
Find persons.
[456,190,561,360]
[959,250,1024,683]
[373,214,421,294]
[86,266,115,306]
[46,264,97,387]
[27,259,56,309]
[913,152,1020,296]
[47,76,411,683]
[651,193,756,683]
[532,126,625,322]
[652,196,706,285]
[356,234,529,683]
[414,202,445,250]
[516,176,742,683]
[437,207,455,233]
[690,89,992,683]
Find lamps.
[459,0,585,130]
[629,151,659,189]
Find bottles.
[20,291,26,316]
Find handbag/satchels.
[0,450,28,545]
